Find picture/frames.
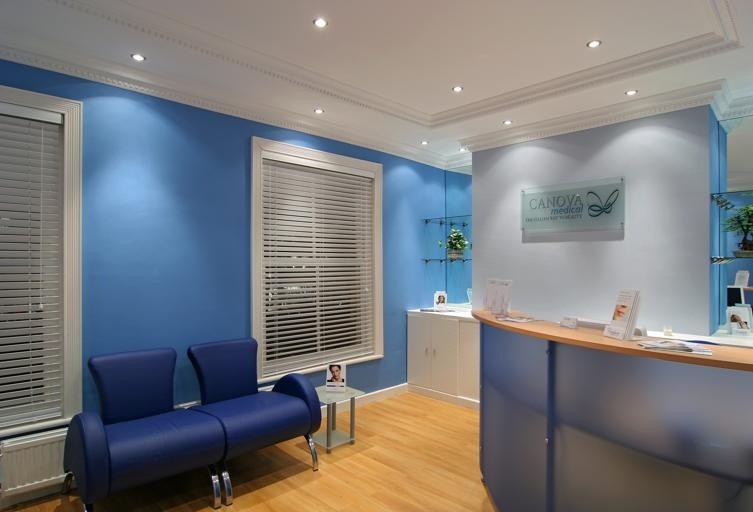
[433,290,448,312]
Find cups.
[663,325,672,336]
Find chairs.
[60,348,223,511]
[185,337,321,506]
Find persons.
[436,294,445,305]
[730,313,750,332]
[326,364,345,383]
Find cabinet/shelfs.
[421,213,472,267]
[406,304,479,408]
[712,189,752,267]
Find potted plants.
[440,228,469,262]
[719,203,752,257]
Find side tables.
[306,382,365,453]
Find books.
[638,339,713,356]
[605,287,642,342]
